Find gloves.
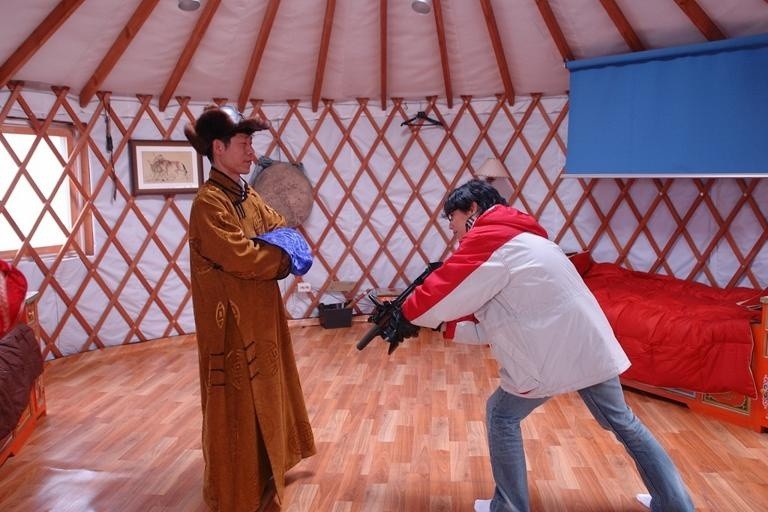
[381,310,418,342]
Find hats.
[182,103,273,156]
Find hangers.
[400,102,442,127]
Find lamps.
[178,0,200,10]
[411,0,431,14]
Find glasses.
[445,209,456,221]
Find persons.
[401,179,697,511]
[182,103,317,511]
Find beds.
[566,247,768,434]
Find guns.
[355,261,444,351]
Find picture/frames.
[128,139,204,195]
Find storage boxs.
[317,303,352,329]
